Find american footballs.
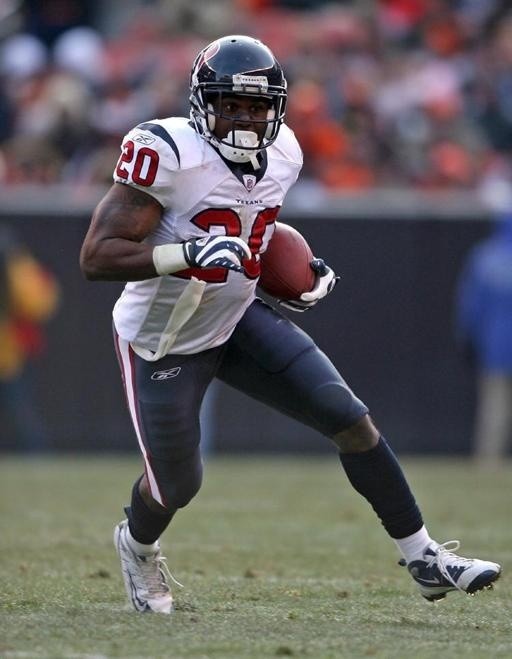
[256,222,316,302]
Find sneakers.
[398,539,501,603]
[113,518,185,614]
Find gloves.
[276,259,341,313]
[183,235,252,275]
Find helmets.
[188,35,288,163]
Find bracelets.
[152,242,190,276]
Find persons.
[1,1,512,211]
[80,35,501,615]
[452,212,512,478]
